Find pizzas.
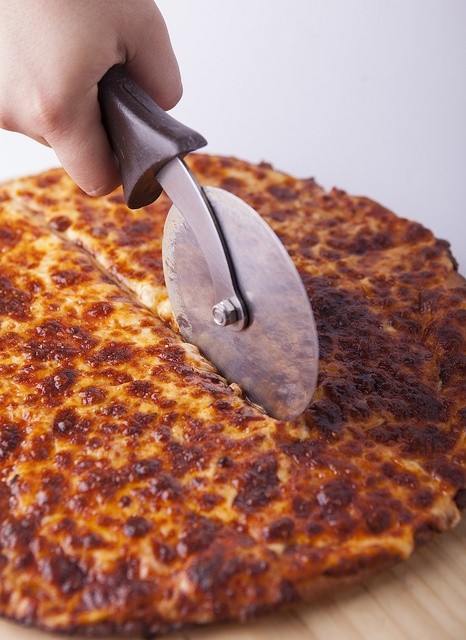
[0,153,465,639]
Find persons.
[0,0,183,197]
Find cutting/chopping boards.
[1,508,466,639]
